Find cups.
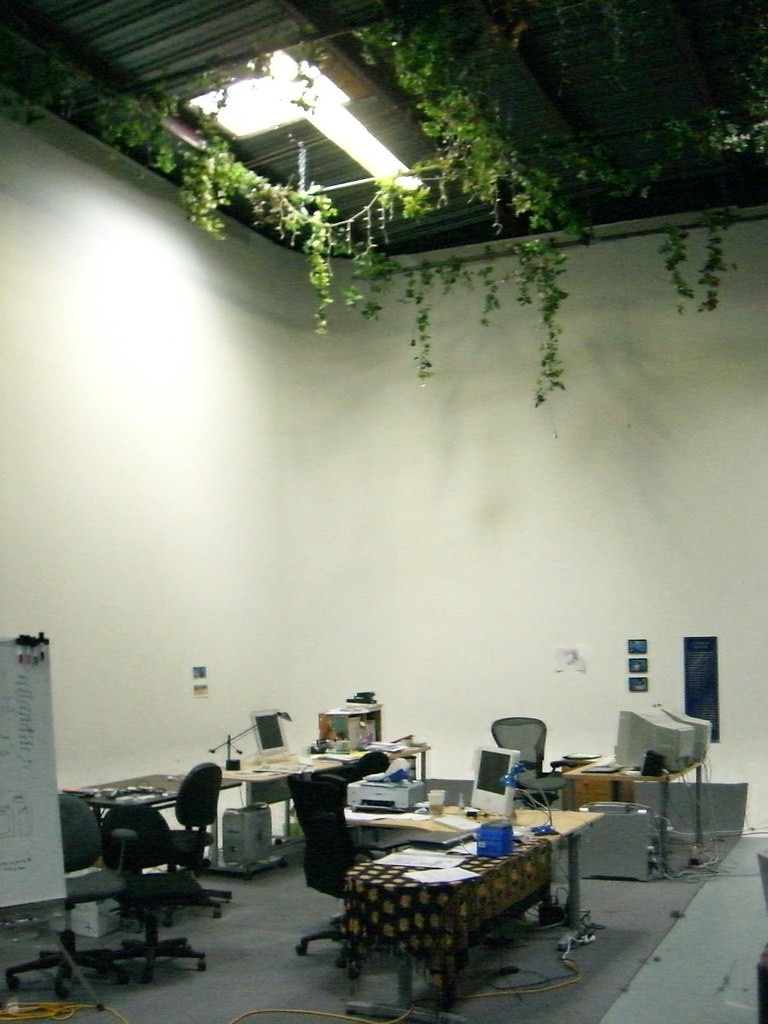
[427,789,445,817]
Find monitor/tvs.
[251,709,288,758]
[471,746,520,823]
[614,703,712,772]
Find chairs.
[490,716,566,808]
[99,802,208,981]
[289,772,393,967]
[5,794,116,997]
[157,763,233,927]
[346,751,387,839]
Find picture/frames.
[629,658,647,673]
[628,639,647,653]
[630,677,648,691]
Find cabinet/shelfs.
[559,763,634,810]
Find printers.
[346,779,425,814]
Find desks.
[275,743,431,852]
[190,760,344,874]
[62,775,244,825]
[560,757,708,876]
[343,809,606,1020]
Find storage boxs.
[66,869,121,935]
[222,804,269,864]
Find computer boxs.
[221,804,273,864]
[578,810,663,882]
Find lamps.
[256,49,429,193]
